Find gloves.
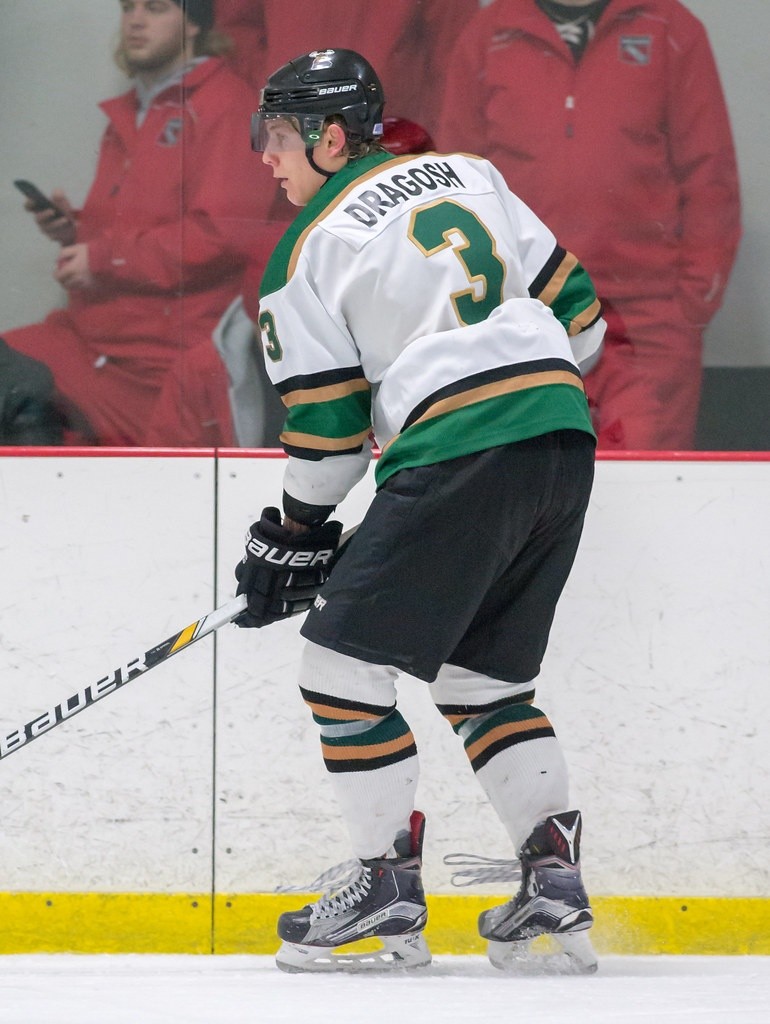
[230,506,343,628]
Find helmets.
[257,47,384,148]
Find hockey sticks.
[0,519,364,762]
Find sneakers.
[274,810,433,974]
[478,810,598,977]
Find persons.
[1,0,745,454]
[235,56,608,971]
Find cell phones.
[15,180,65,222]
